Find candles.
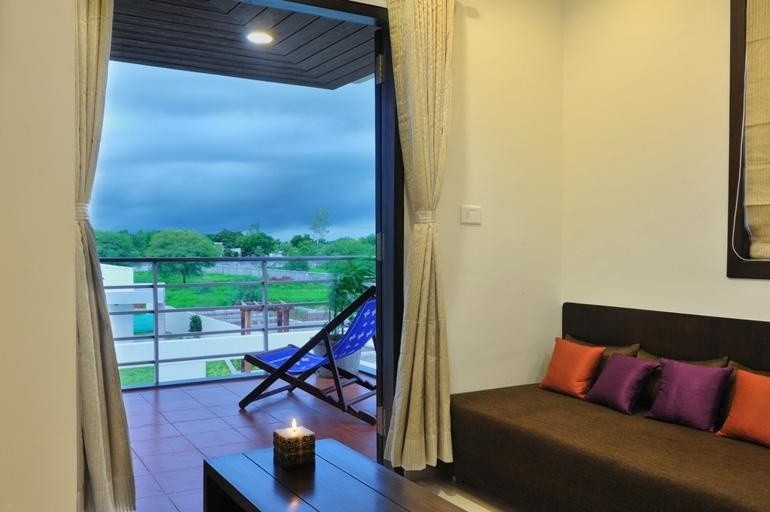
[272,418,315,472]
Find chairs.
[238,286,378,425]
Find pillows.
[582,352,660,415]
[714,370,770,447]
[637,350,728,409]
[718,360,770,428]
[563,334,640,386]
[539,336,607,400]
[642,359,733,433]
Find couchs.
[442,302,770,512]
